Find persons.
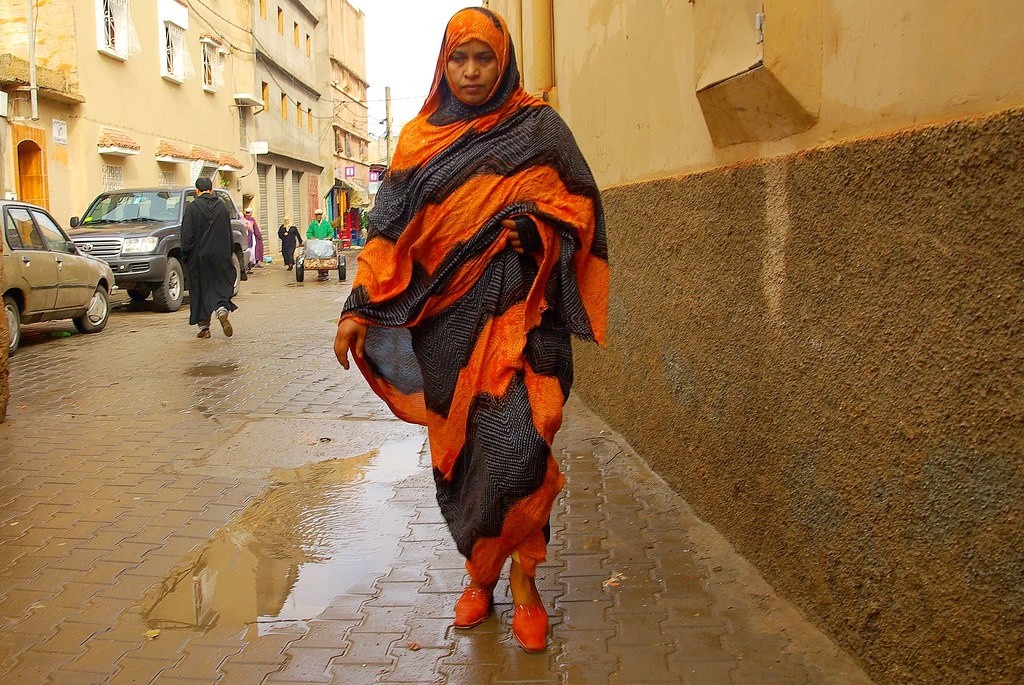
[238,208,264,275]
[306,209,335,281]
[333,7,610,652]
[278,216,302,270]
[179,177,238,337]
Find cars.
[0,199,118,357]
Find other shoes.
[454,572,493,629]
[197,330,210,338]
[288,266,293,270]
[219,312,233,337]
[319,274,328,277]
[507,577,549,654]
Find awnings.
[335,178,370,209]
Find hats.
[246,208,252,212]
[315,209,323,214]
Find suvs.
[65,187,251,313]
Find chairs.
[30,224,53,249]
[175,201,190,217]
[123,204,142,218]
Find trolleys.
[296,239,346,281]
